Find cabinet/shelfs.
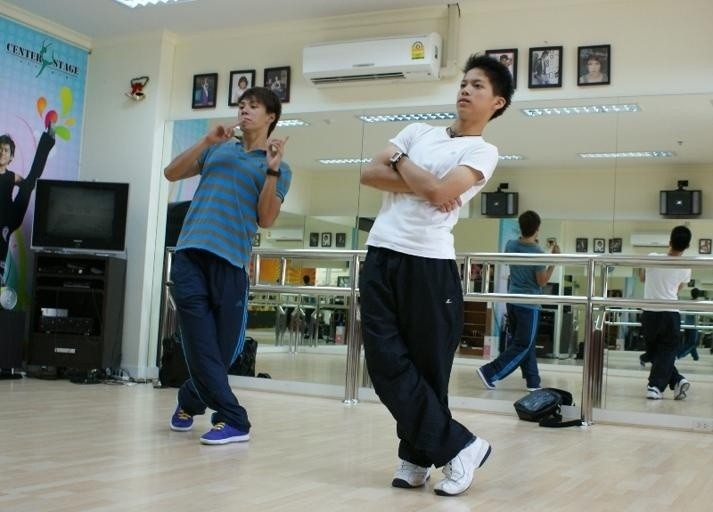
[26,251,128,383]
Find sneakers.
[199,421,250,445]
[674,376,690,400]
[392,460,430,489]
[169,401,194,431]
[433,436,491,496]
[647,384,663,399]
[476,366,496,390]
[526,382,543,392]
[47,121,55,139]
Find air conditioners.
[302,31,443,87]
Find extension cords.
[112,374,129,380]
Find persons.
[0,121,55,287]
[639,226,692,401]
[164,86,292,445]
[201,77,209,106]
[266,78,273,89]
[235,76,249,102]
[579,54,608,83]
[476,210,561,392]
[359,54,513,497]
[500,55,514,68]
[271,75,283,99]
[534,51,550,85]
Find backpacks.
[228,337,270,379]
[153,336,189,389]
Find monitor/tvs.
[481,191,518,215]
[29,179,130,255]
[659,190,702,215]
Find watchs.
[389,151,409,171]
[266,168,281,177]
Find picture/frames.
[192,72,218,107]
[485,48,517,88]
[528,46,563,87]
[263,66,291,102]
[578,44,611,86]
[228,70,256,106]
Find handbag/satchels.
[542,388,572,406]
[514,388,582,427]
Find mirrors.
[155,94,713,420]
